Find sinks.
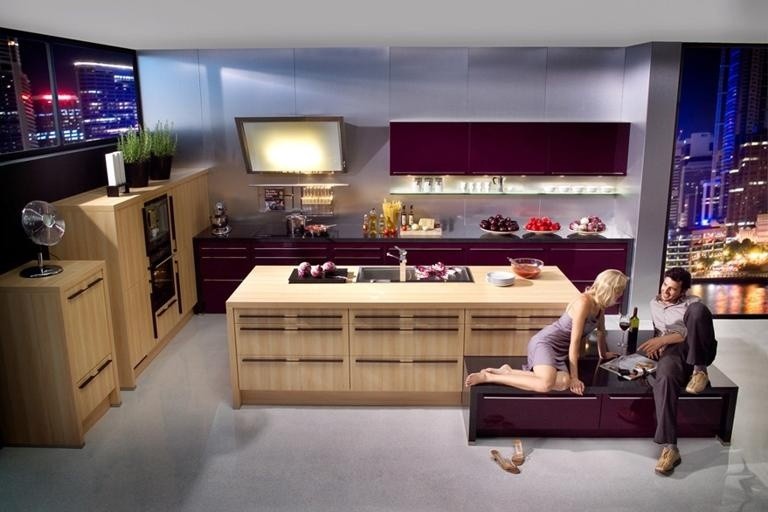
[356,266,474,282]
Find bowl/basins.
[546,186,612,192]
[486,272,516,287]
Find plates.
[525,228,557,235]
[569,228,605,236]
[599,354,658,381]
[479,225,521,236]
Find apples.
[569,216,605,231]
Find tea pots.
[493,176,505,192]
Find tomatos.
[526,217,560,231]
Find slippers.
[491,450,520,475]
[512,439,524,466]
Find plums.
[480,214,519,231]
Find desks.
[462,356,738,442]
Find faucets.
[386,245,409,281]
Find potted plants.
[117,123,152,188]
[148,120,177,180]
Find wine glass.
[619,315,630,347]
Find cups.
[464,180,492,192]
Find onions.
[321,261,336,274]
[298,262,310,278]
[310,264,322,278]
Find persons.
[464,268,630,396]
[636,267,717,477]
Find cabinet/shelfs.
[384,242,467,266]
[193,237,252,314]
[468,123,547,196]
[0,260,121,448]
[467,242,548,266]
[390,122,469,194]
[47,168,210,390]
[253,242,330,265]
[547,122,631,194]
[548,242,630,314]
[330,241,384,264]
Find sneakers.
[655,447,681,476]
[686,369,709,394]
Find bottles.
[362,205,414,234]
[626,307,639,354]
[303,185,334,214]
[414,177,443,192]
